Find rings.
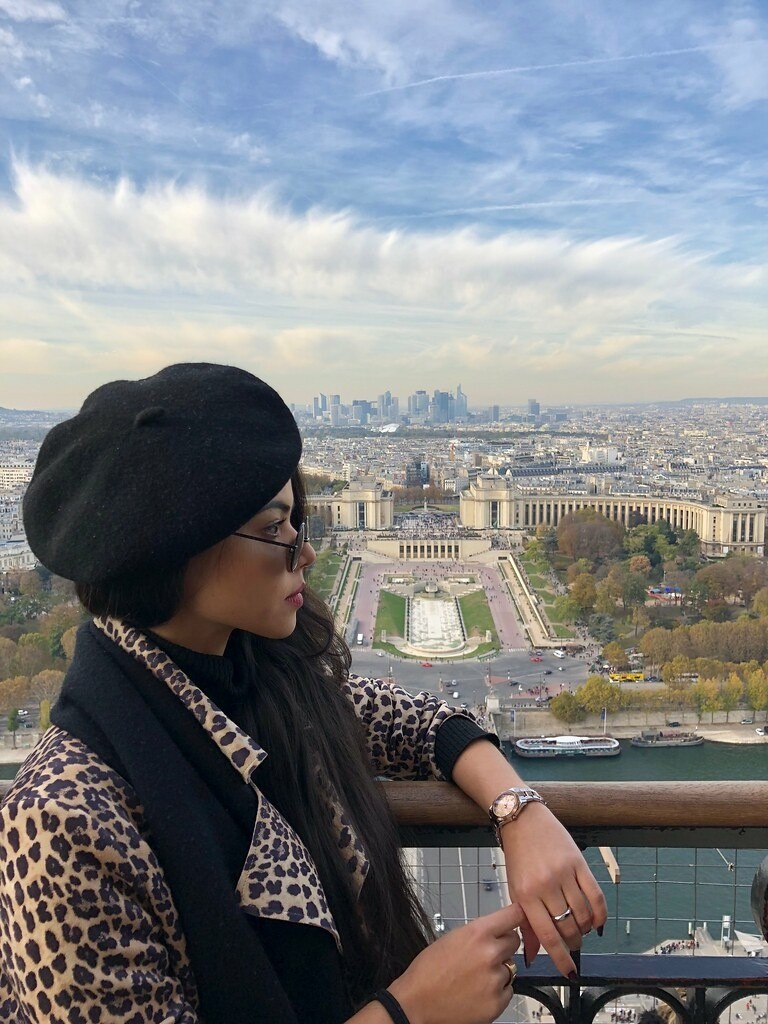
[552,907,572,922]
[504,960,520,979]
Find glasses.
[231,515,308,573]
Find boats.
[514,734,622,758]
[629,726,704,747]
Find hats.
[22,362,303,630]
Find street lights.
[511,709,515,738]
[602,707,607,735]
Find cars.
[739,718,768,736]
[348,625,703,709]
[429,877,494,933]
[669,721,679,727]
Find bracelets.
[375,988,410,1024]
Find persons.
[0,361,609,1024]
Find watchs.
[487,787,548,851]
